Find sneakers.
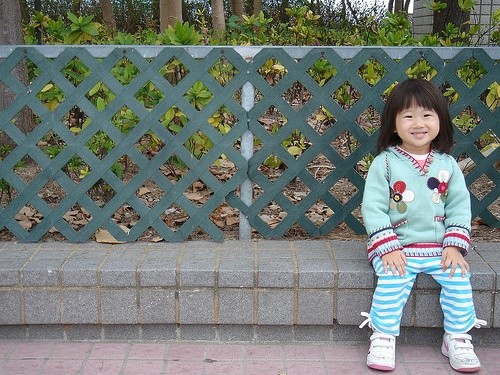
[441,330,481,372]
[367,329,397,371]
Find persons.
[361,79,481,372]
